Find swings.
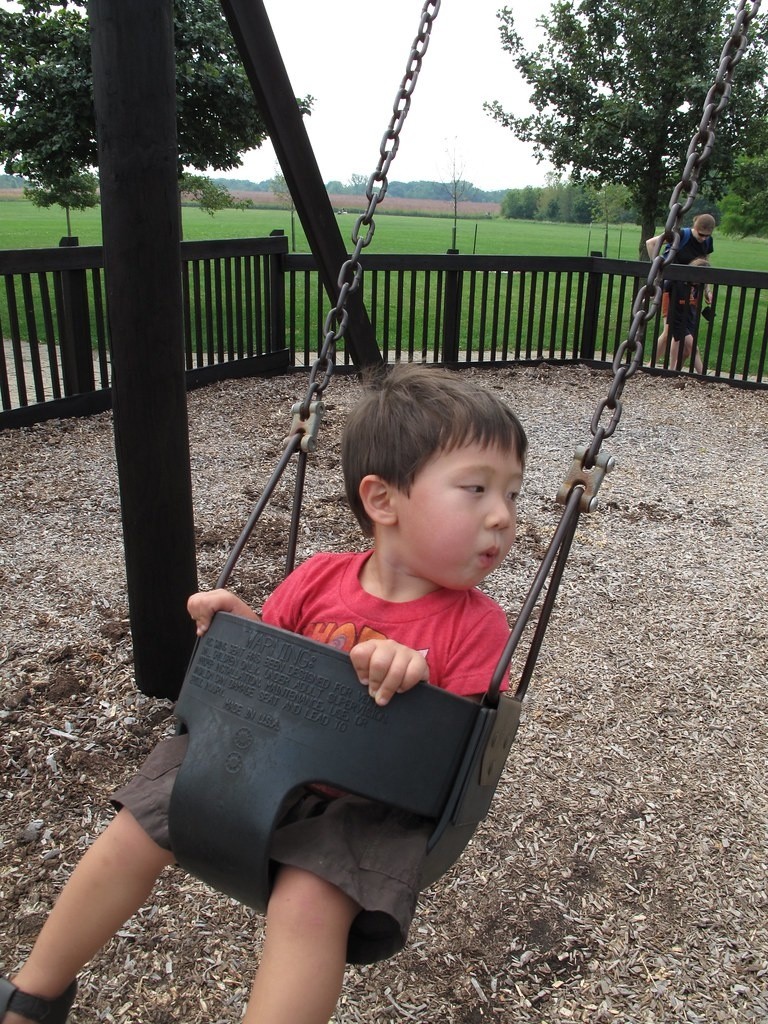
[157,2,764,921]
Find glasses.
[696,230,711,238]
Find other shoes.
[0,977,78,1024]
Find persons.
[0,365,528,1024]
[646,214,715,374]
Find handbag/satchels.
[702,306,716,321]
